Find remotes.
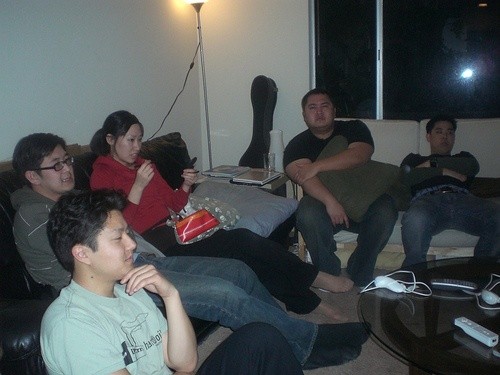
[430,278,478,293]
[452,317,498,348]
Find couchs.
[297,118,500,274]
[0,133,290,374]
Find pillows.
[310,135,402,223]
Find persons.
[87,110,354,322]
[37,186,305,374]
[8,133,372,375]
[399,115,500,267]
[282,91,400,285]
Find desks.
[358,253,500,374]
[201,165,288,191]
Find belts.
[431,186,458,194]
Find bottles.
[268,129,286,172]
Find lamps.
[177,0,213,173]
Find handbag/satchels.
[168,206,220,246]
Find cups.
[263,151,276,175]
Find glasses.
[33,156,74,171]
